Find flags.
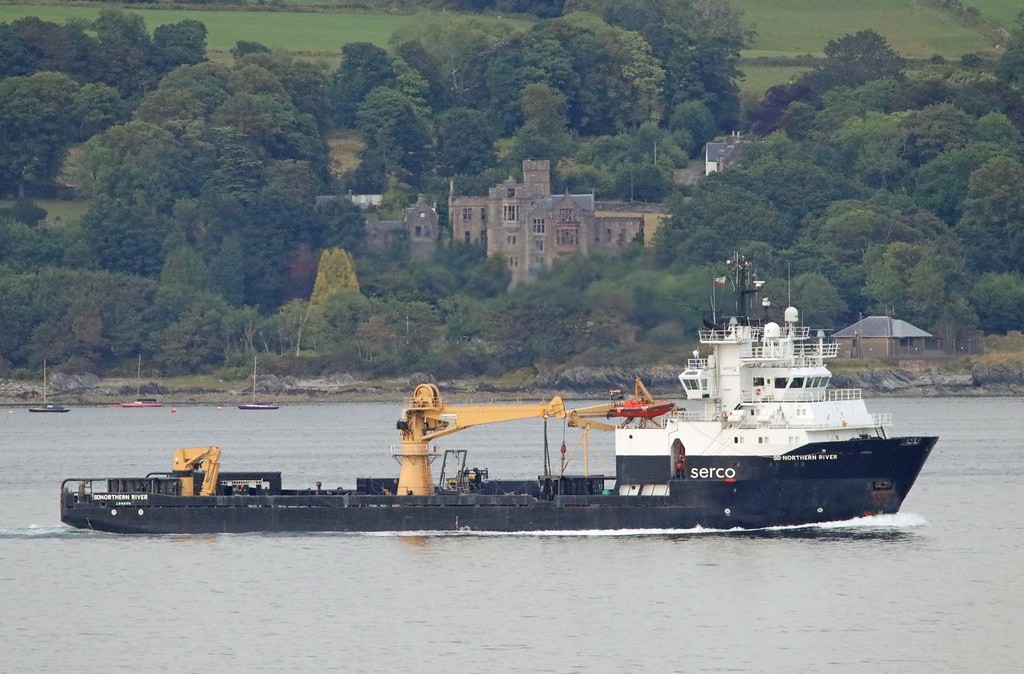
[714,276,726,284]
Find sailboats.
[237,356,281,410]
[119,355,164,408]
[27,359,72,414]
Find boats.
[604,388,675,419]
[58,252,941,536]
